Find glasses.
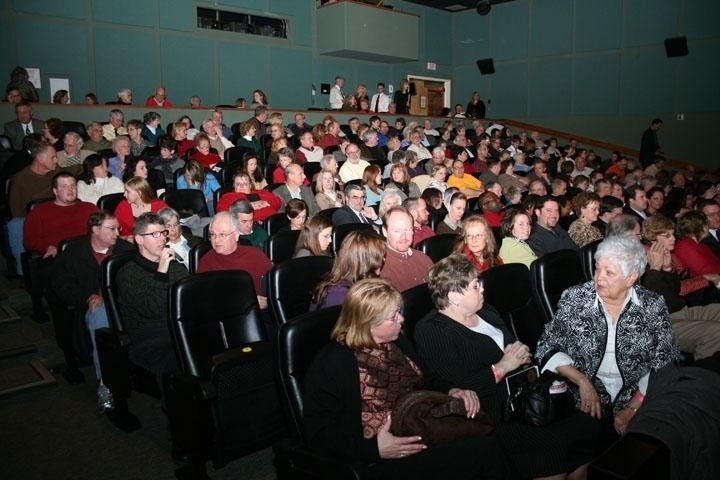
[483,149,488,151]
[139,230,169,238]
[463,234,487,240]
[200,146,210,150]
[484,196,501,204]
[13,95,20,97]
[656,234,676,240]
[98,223,123,233]
[462,279,484,292]
[347,196,366,201]
[454,167,464,170]
[635,232,646,237]
[208,229,239,241]
[705,213,720,218]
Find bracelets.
[625,403,638,413]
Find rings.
[585,404,592,408]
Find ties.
[716,229,719,240]
[26,124,30,136]
[115,127,119,136]
[375,94,379,114]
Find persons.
[0,75,718,366]
[120,212,196,467]
[410,255,593,478]
[22,171,102,298]
[155,207,193,264]
[301,276,512,478]
[535,232,682,438]
[309,229,389,311]
[226,197,271,248]
[199,211,277,311]
[53,209,133,413]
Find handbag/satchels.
[503,365,541,425]
[390,389,496,448]
[520,369,576,430]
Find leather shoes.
[171,450,189,463]
[62,364,82,387]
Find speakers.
[475,58,495,74]
[663,35,689,58]
